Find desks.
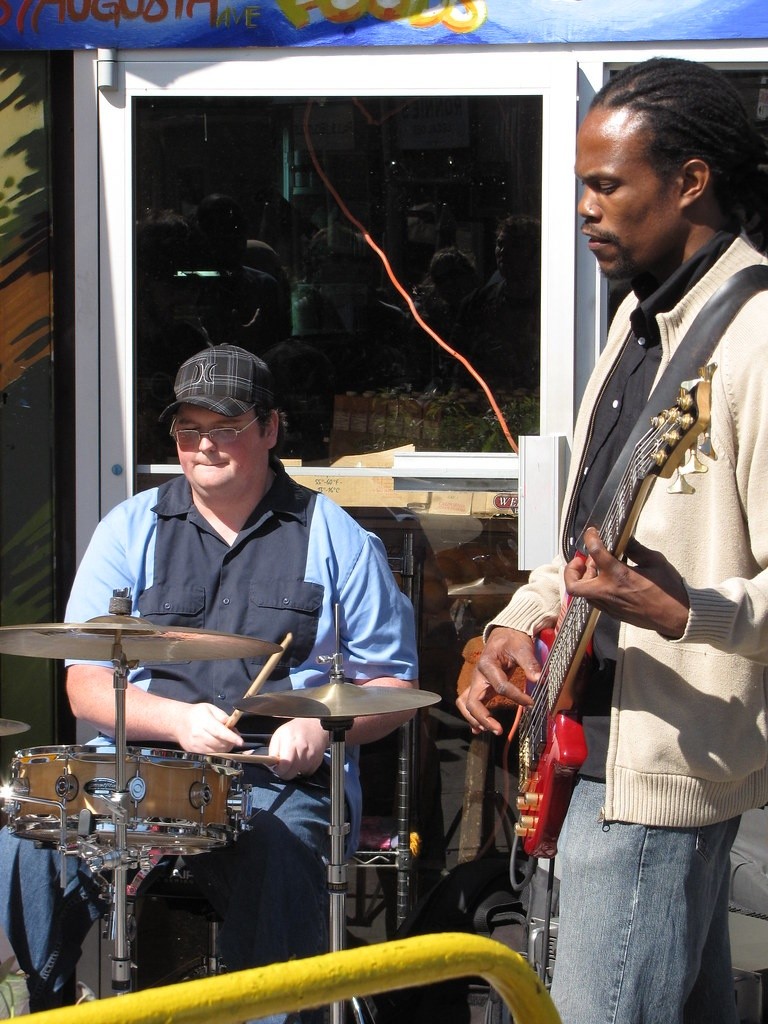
[448,577,528,863]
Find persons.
[0,343,418,1024]
[138,195,540,459]
[455,56,767,1023]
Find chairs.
[340,504,426,940]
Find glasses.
[167,416,260,443]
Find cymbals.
[229,678,446,720]
[0,717,31,737]
[1,614,285,666]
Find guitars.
[510,364,719,857]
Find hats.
[156,341,283,436]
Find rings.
[298,770,305,776]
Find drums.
[11,745,244,857]
